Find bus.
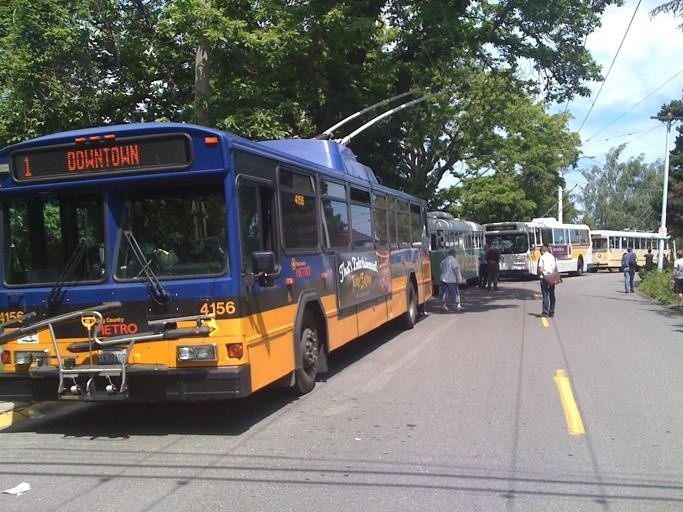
[482,217,593,277]
[589,228,676,272]
[426,210,485,293]
[0,122,432,402]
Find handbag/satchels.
[544,272,561,285]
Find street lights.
[555,155,596,224]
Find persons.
[622,247,637,293]
[479,242,500,290]
[536,246,558,317]
[644,249,653,264]
[673,250,683,311]
[439,249,465,312]
